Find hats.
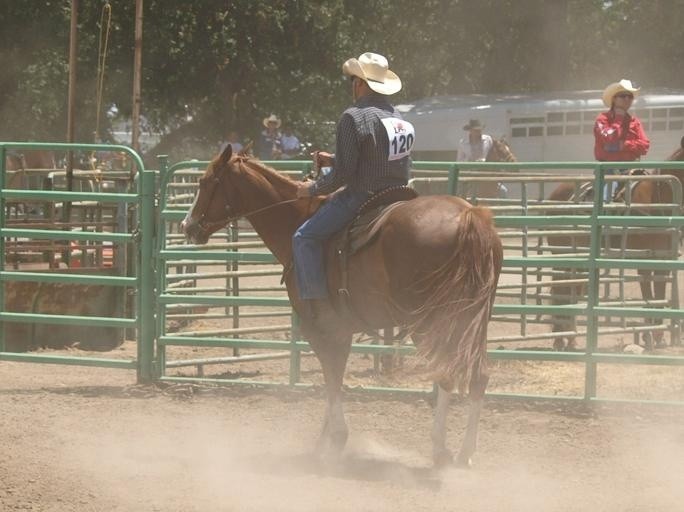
[602,79,641,107]
[343,52,402,95]
[463,119,486,130]
[263,115,281,128]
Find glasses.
[615,94,635,98]
[351,76,355,81]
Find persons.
[594,79,650,247]
[457,116,508,198]
[259,112,301,162]
[291,53,411,334]
[220,129,243,155]
